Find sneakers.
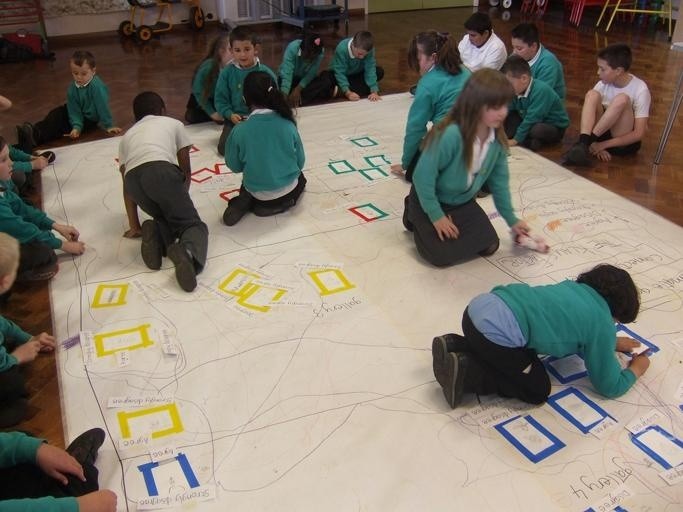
[572,142,597,168]
[15,121,56,166]
[165,238,197,294]
[139,217,163,272]
[219,187,256,227]
[15,262,61,283]
[430,333,465,396]
[558,141,580,168]
[57,426,107,496]
[402,194,414,234]
[443,350,472,409]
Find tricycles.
[118,0,214,42]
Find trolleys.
[489,0,549,9]
[274,0,348,38]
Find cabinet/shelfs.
[273,0,351,34]
[215,0,326,30]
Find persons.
[432,263,651,408]
[119,91,209,292]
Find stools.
[519,0,682,167]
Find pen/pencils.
[71,232,73,241]
[63,134,71,137]
[629,347,651,364]
[47,152,53,160]
[242,118,247,119]
